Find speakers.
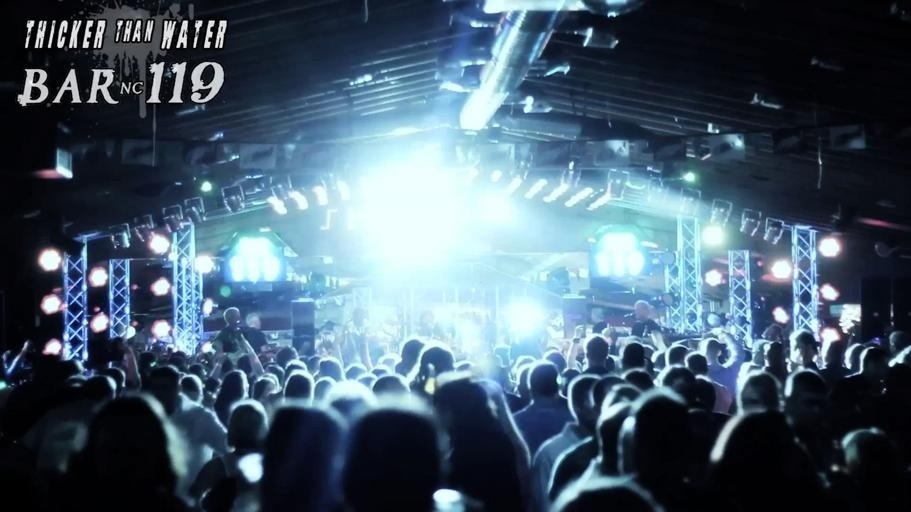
[292,299,315,336]
[564,296,587,340]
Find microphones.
[624,313,636,319]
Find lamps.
[494,152,619,212]
[261,164,370,217]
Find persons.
[1,299,911,511]
